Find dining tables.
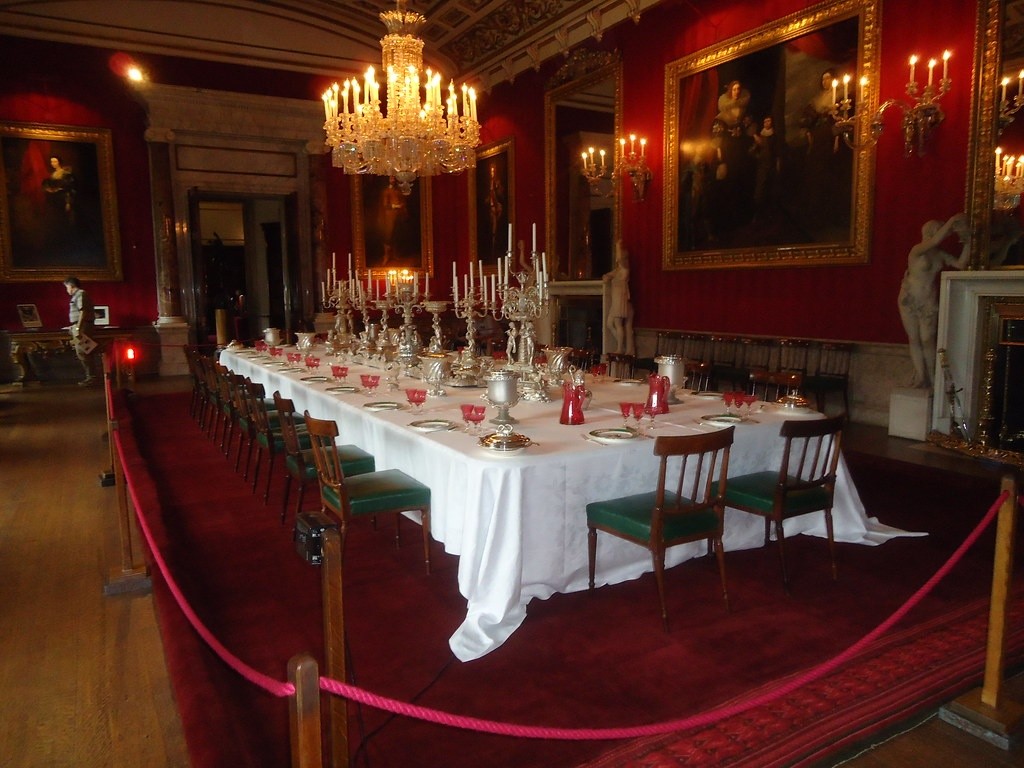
[219,341,929,663]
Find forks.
[694,419,722,430]
[582,434,606,446]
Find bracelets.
[74,327,80,331]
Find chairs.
[585,425,736,635]
[707,411,847,590]
[489,323,859,423]
[176,342,432,573]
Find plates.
[410,419,456,432]
[701,413,746,423]
[300,376,329,383]
[476,442,534,456]
[262,361,287,367]
[327,386,360,395]
[235,350,257,354]
[619,401,659,429]
[364,402,404,412]
[247,355,269,360]
[277,368,306,373]
[589,429,638,440]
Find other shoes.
[77,376,101,387]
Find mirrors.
[959,0,1024,271]
[539,43,623,296]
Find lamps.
[995,66,1024,135]
[989,145,1024,213]
[581,145,607,196]
[619,131,651,201]
[322,0,483,195]
[901,45,953,158]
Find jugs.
[559,381,586,425]
[645,374,670,415]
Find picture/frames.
[0,121,124,283]
[346,165,433,280]
[661,0,881,270]
[466,133,516,278]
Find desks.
[7,326,155,383]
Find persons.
[328,309,535,363]
[604,240,636,356]
[517,239,539,274]
[898,214,972,389]
[522,321,535,368]
[504,322,518,367]
[63,277,96,386]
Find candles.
[322,223,550,309]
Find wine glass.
[462,402,486,436]
[406,389,427,415]
[723,391,756,417]
[332,365,348,385]
[591,361,609,384]
[253,341,320,374]
[360,374,381,395]
[492,352,508,363]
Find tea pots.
[226,339,244,351]
